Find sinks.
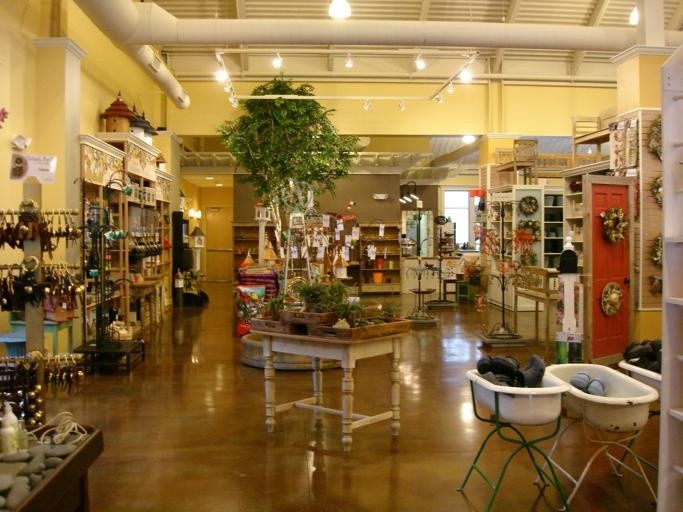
[545,359,660,435]
[618,354,662,398]
[467,365,567,426]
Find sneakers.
[570,371,606,394]
[477,354,545,387]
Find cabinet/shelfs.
[230,221,279,296]
[362,210,486,307]
[487,183,588,343]
[78,131,174,354]
[656,43,683,511]
[74,340,145,376]
[172,211,193,271]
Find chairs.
[495,139,538,185]
[570,116,610,168]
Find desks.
[9,318,73,398]
[249,329,411,453]
[0,420,103,511]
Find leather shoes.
[623,339,661,373]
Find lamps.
[174,268,185,307]
[328,0,352,18]
[188,207,195,223]
[196,209,201,220]
[214,47,480,111]
[399,181,424,209]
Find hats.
[21,256,39,272]
[18,199,38,212]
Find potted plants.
[251,295,307,334]
[279,274,379,325]
[316,298,414,340]
